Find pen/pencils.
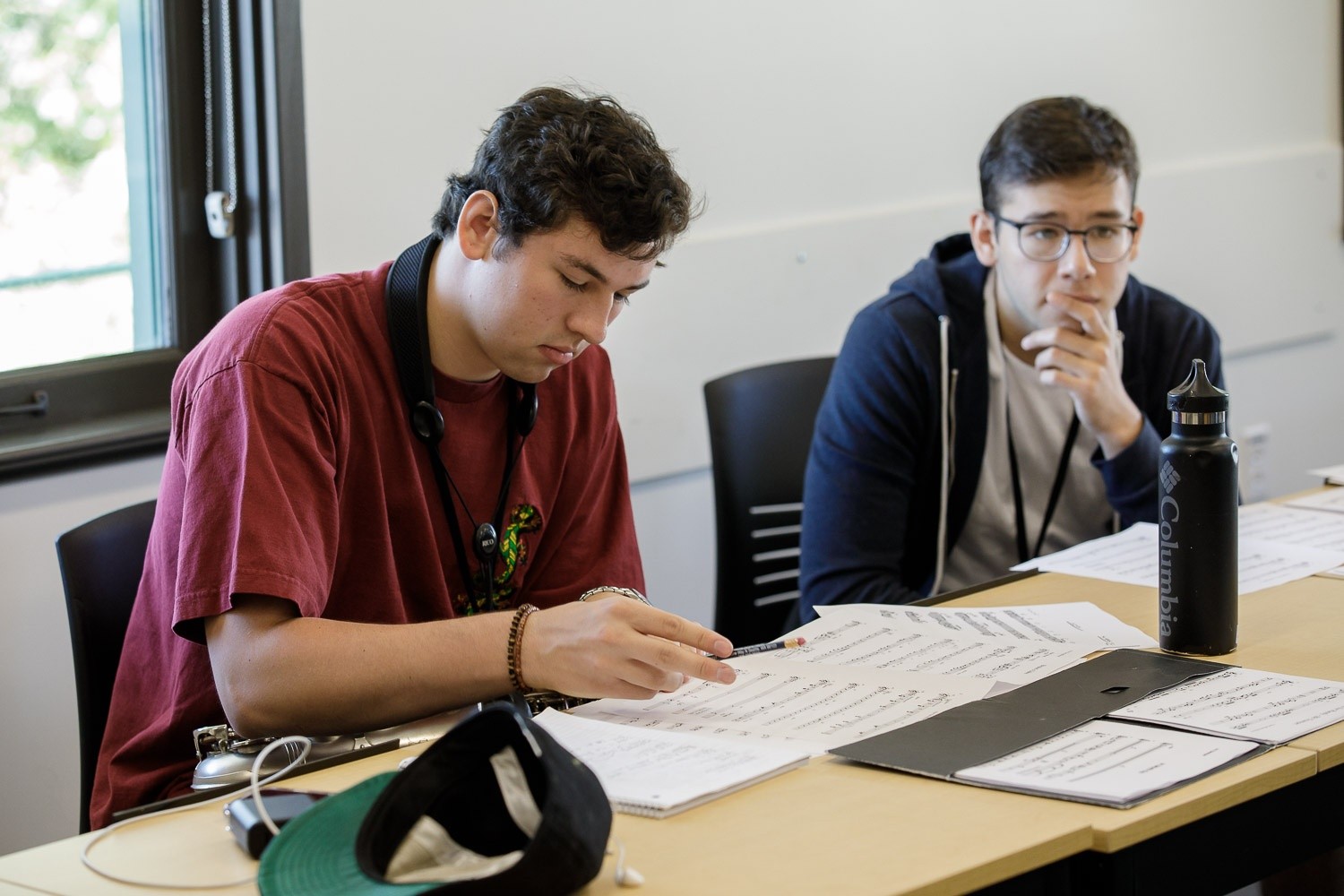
[706,637,805,660]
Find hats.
[257,700,612,896]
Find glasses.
[989,211,1138,265]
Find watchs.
[580,585,654,607]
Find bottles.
[1159,359,1239,657]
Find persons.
[799,96,1241,624]
[90,88,737,830]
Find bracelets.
[507,603,539,696]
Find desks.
[0,484,1344,895]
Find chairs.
[54,498,159,833]
[703,356,838,648]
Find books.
[510,708,812,820]
